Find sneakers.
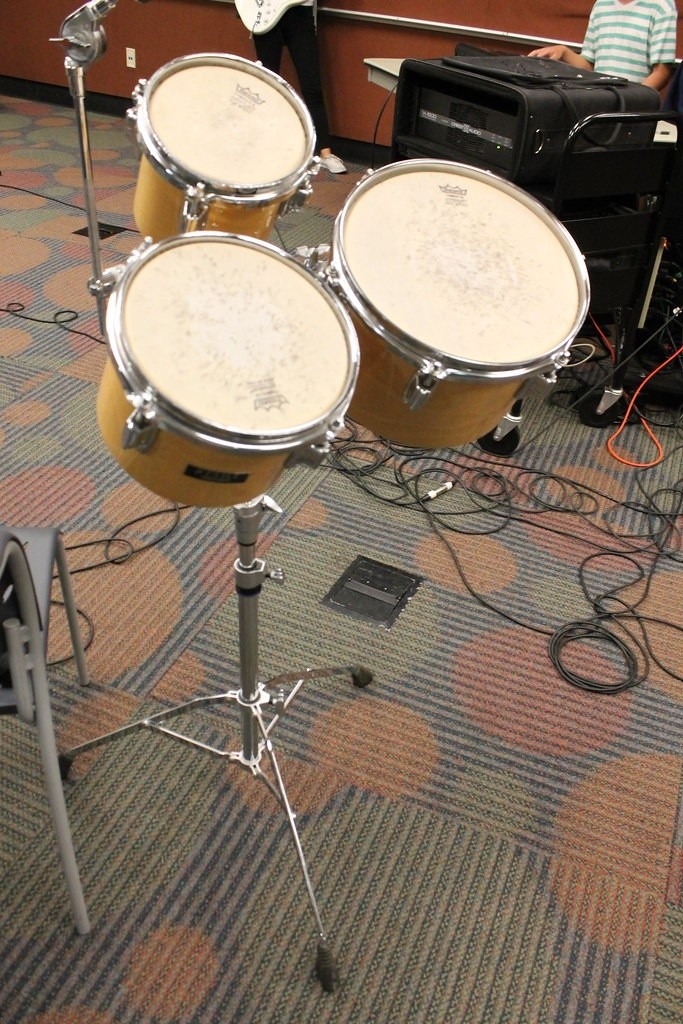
[318,154,347,174]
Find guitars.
[232,0,309,34]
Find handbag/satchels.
[441,54,631,148]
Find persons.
[527,0,678,91]
[235,0,347,173]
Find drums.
[127,51,321,240]
[95,226,362,509]
[320,158,592,450]
